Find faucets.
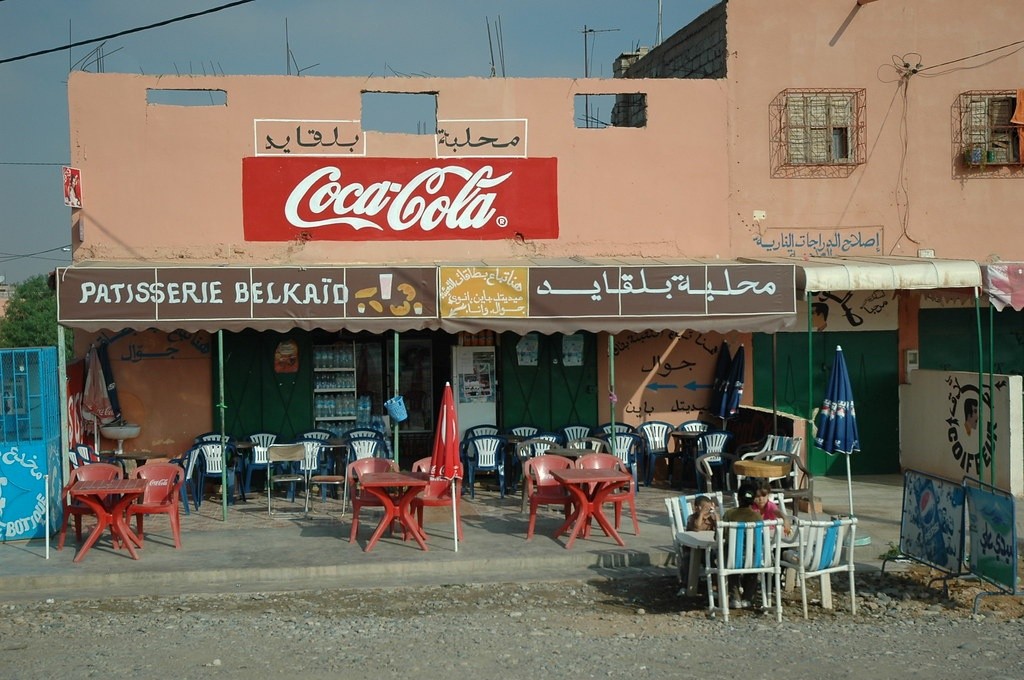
[110,408,124,428]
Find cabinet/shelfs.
[313,367,356,421]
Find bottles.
[315,393,356,416]
[357,396,393,460]
[458,329,493,346]
[316,373,355,388]
[386,465,398,494]
[314,347,354,368]
[318,422,355,439]
[350,449,355,461]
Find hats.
[738,484,756,505]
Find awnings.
[54,254,1024,338]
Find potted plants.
[965,144,986,174]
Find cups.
[379,273,393,299]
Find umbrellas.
[79,340,121,455]
[814,344,860,517]
[709,339,745,431]
[428,381,464,553]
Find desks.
[362,472,430,553]
[115,452,167,460]
[550,469,631,550]
[675,524,827,596]
[671,432,701,489]
[733,460,790,490]
[70,479,149,563]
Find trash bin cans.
[384,396,407,422]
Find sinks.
[100,425,141,439]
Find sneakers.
[729,599,752,608]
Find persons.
[676,496,721,596]
[702,483,764,608]
[750,477,790,538]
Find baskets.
[384,396,408,422]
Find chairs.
[459,422,642,499]
[525,454,584,540]
[678,420,718,432]
[737,435,803,504]
[694,430,733,491]
[753,450,817,520]
[705,518,783,623]
[169,429,389,520]
[768,517,858,620]
[636,421,679,487]
[732,492,788,516]
[575,453,640,539]
[348,458,407,544]
[69,444,126,503]
[665,491,724,588]
[405,456,463,544]
[695,452,741,492]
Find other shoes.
[677,588,687,597]
[713,592,719,599]
